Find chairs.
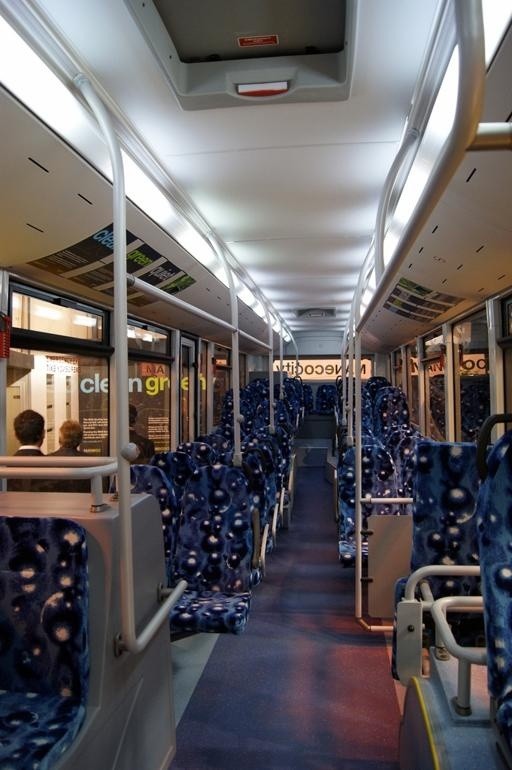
[326,374,511,769]
[0,374,303,770]
[303,383,313,414]
[314,384,339,414]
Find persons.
[48,419,92,455]
[101,403,158,457]
[9,409,48,457]
